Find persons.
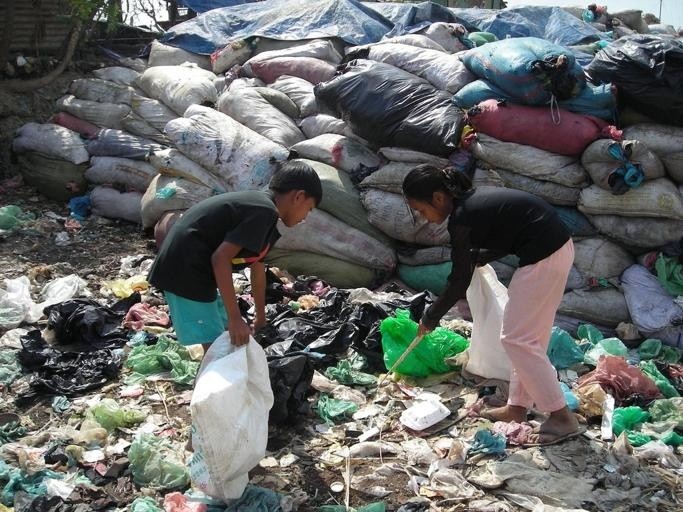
[146,161,323,355]
[402,163,587,448]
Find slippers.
[524,425,586,446]
